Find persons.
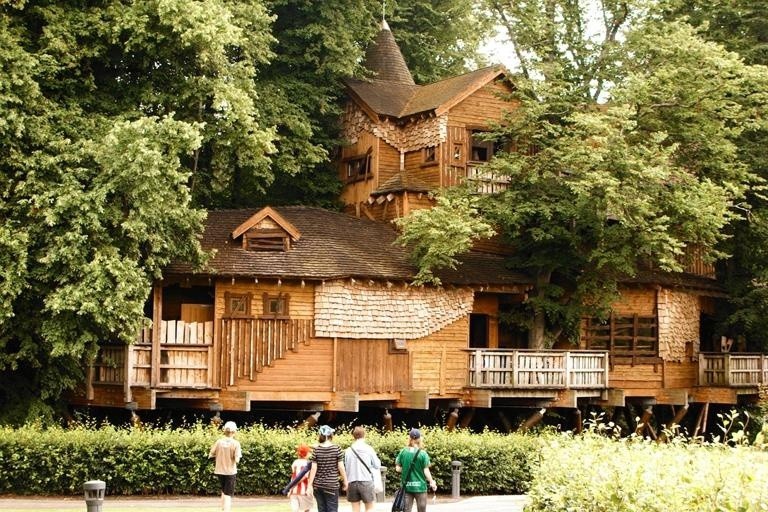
[395,428,438,511]
[303,425,349,511]
[208,421,242,511]
[342,426,382,512]
[287,445,317,512]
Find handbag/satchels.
[390,486,407,512]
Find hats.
[320,425,335,436]
[409,428,421,440]
[223,420,238,432]
